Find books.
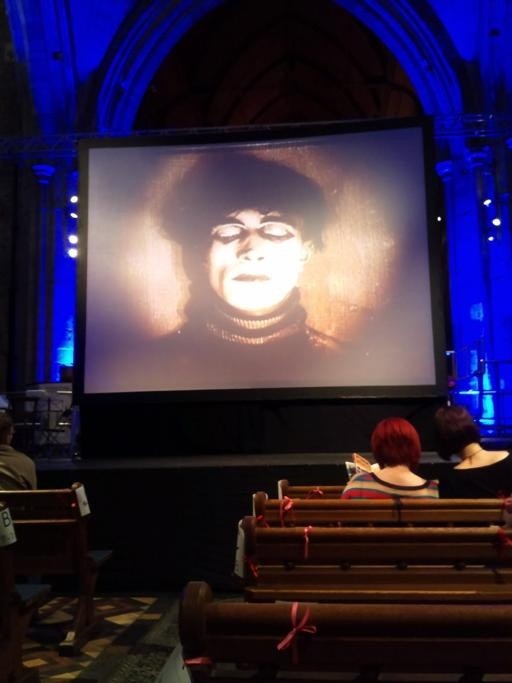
[345,451,381,479]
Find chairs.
[6,383,74,455]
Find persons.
[0,408,69,644]
[118,146,382,387]
[433,403,511,498]
[339,415,440,501]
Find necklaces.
[463,448,484,464]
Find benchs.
[277,479,344,500]
[176,582,511,682]
[233,517,510,603]
[2,482,113,656]
[252,491,511,527]
[2,502,52,680]
